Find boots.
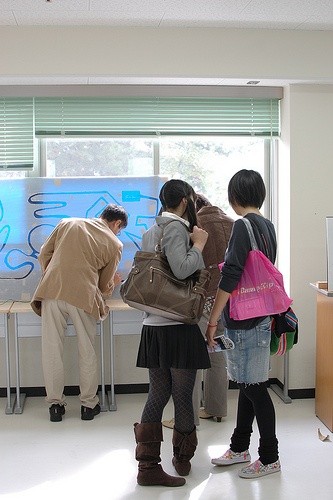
[133,421,186,488]
[172,423,198,477]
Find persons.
[133,178,210,488]
[206,169,282,479]
[160,193,236,431]
[31,203,128,422]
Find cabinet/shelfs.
[310,280,333,432]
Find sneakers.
[80,404,102,420]
[49,403,66,422]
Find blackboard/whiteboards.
[0,175,174,301]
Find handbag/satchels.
[217,217,286,321]
[269,305,299,358]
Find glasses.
[119,219,212,323]
[116,226,121,236]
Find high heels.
[198,407,221,422]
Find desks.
[0,299,209,415]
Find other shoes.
[211,447,251,465]
[237,458,281,479]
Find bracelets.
[206,321,218,328]
